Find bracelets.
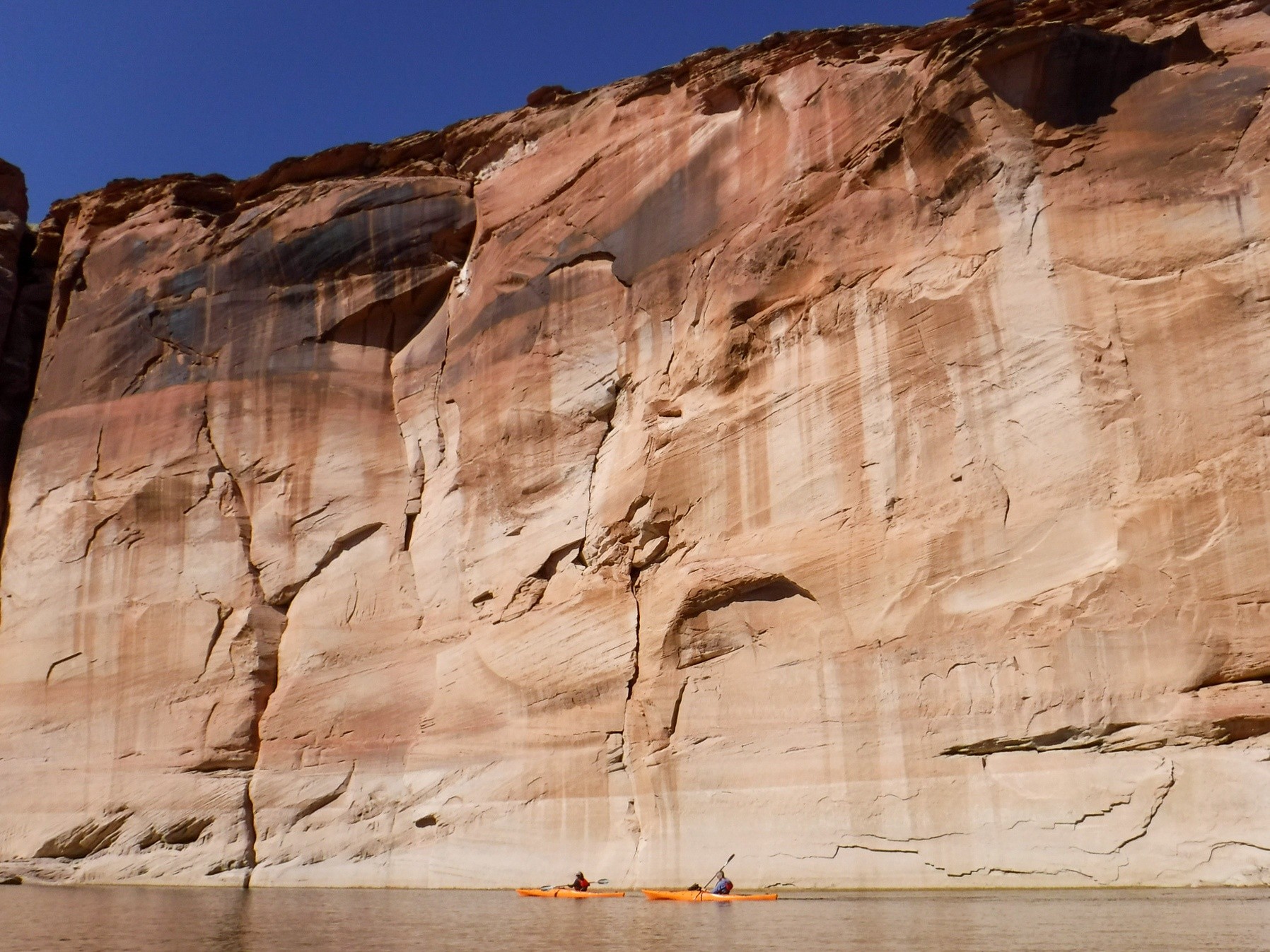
[705,889,707,891]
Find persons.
[554,871,590,892]
[697,871,730,894]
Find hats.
[576,871,581,875]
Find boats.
[640,883,780,902]
[514,886,625,898]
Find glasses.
[717,875,721,878]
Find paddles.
[540,878,609,891]
[691,854,735,900]
